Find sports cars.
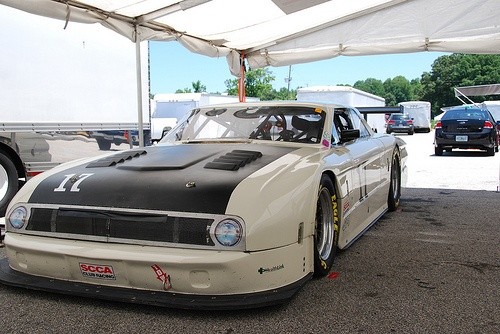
[0,97,408,312]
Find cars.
[386,113,415,135]
[434,107,500,156]
